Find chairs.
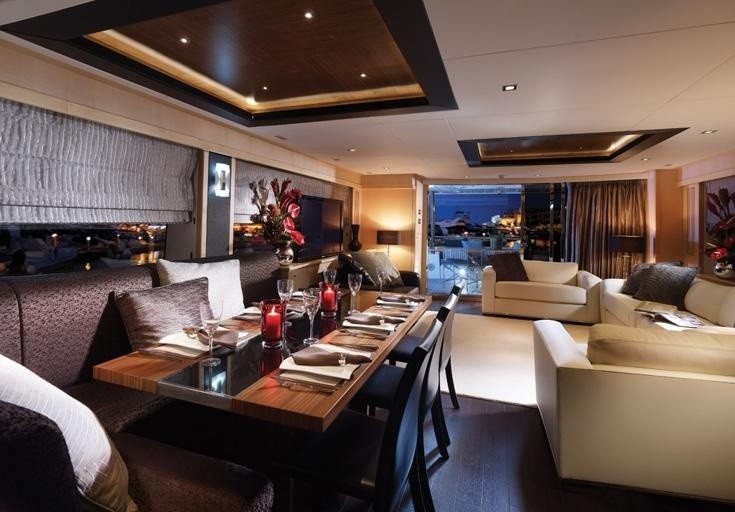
[441,246,468,288]
[481,260,603,324]
[466,246,489,292]
[338,253,421,295]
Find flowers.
[260,178,305,254]
[703,188,735,266]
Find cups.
[260,299,286,349]
[320,283,338,317]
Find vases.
[348,224,361,252]
[713,259,734,279]
[274,240,294,266]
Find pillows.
[632,261,701,311]
[115,277,214,352]
[157,258,245,320]
[351,251,404,288]
[1,356,139,512]
[619,259,683,295]
[487,251,530,281]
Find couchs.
[600,277,735,329]
[534,320,734,503]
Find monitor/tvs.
[298,195,344,263]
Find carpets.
[384,310,593,409]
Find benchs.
[0,248,292,512]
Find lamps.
[377,230,401,256]
[608,234,645,280]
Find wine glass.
[302,288,322,345]
[322,256,338,286]
[375,264,387,296]
[278,279,294,327]
[348,273,363,315]
[198,300,224,367]
